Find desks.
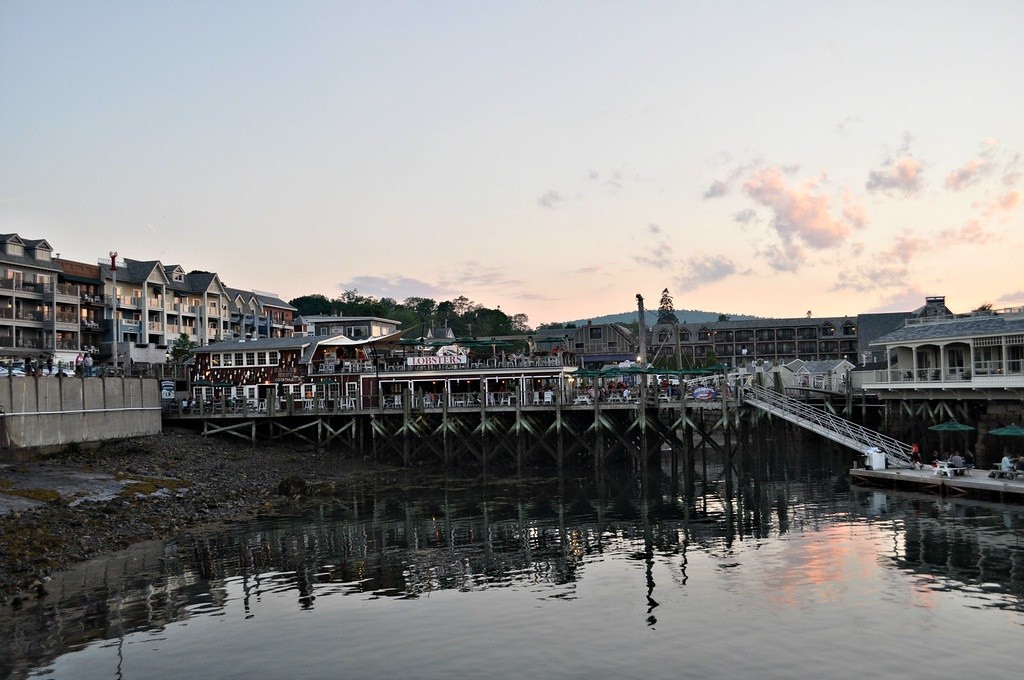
[487,358,495,367]
[857,455,869,470]
[386,398,394,406]
[993,463,1017,481]
[303,399,325,412]
[936,460,972,477]
[658,393,671,403]
[628,394,638,404]
[515,356,530,367]
[608,393,623,403]
[337,396,350,410]
[574,395,592,406]
[547,356,558,366]
[403,361,411,371]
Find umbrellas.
[569,362,735,377]
[927,418,974,448]
[987,422,1024,455]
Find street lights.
[166,350,170,372]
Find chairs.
[16,283,102,354]
[451,393,481,408]
[416,397,425,408]
[488,391,554,405]
[961,368,971,380]
[931,369,941,380]
[382,395,403,409]
[906,371,912,381]
[918,370,926,381]
[437,394,443,408]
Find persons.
[909,442,921,469]
[1000,453,1020,474]
[950,451,965,470]
[181,352,558,414]
[652,374,659,385]
[6,352,99,381]
[570,378,732,407]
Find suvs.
[657,375,687,387]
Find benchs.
[990,470,1024,481]
[932,466,976,478]
[653,397,672,403]
[573,398,594,405]
[606,397,624,403]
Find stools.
[259,402,267,411]
[349,398,356,410]
[318,360,560,374]
[425,402,433,407]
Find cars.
[0,364,125,376]
